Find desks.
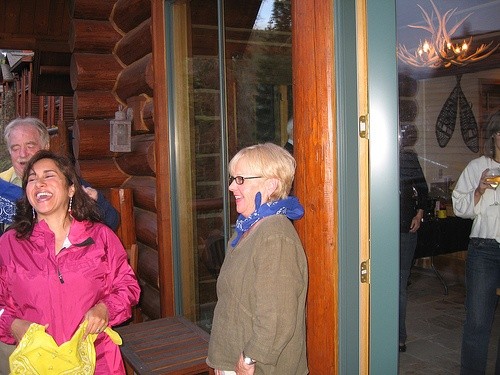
[411,215,473,296]
[112,314,214,375]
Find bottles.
[433,197,442,220]
[437,205,448,221]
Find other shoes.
[399,344,406,352]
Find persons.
[0,150,141,375]
[0,118,121,237]
[284,118,294,157]
[399,150,429,351]
[205,142,310,375]
[451,108,500,375]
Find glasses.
[229,176,261,185]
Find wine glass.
[485,168,500,206]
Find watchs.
[243,350,256,365]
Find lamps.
[109,111,132,153]
[396,0,500,70]
